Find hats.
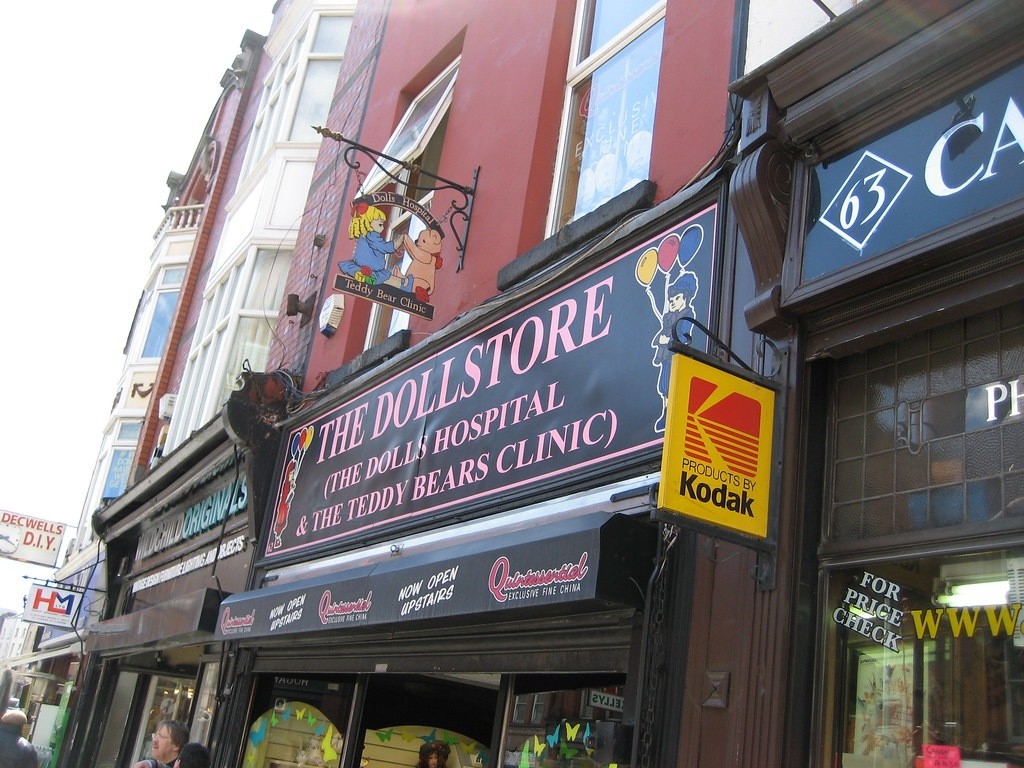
[1,709,29,725]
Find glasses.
[151,733,174,741]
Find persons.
[0,710,38,768]
[416,741,450,768]
[135,719,212,768]
